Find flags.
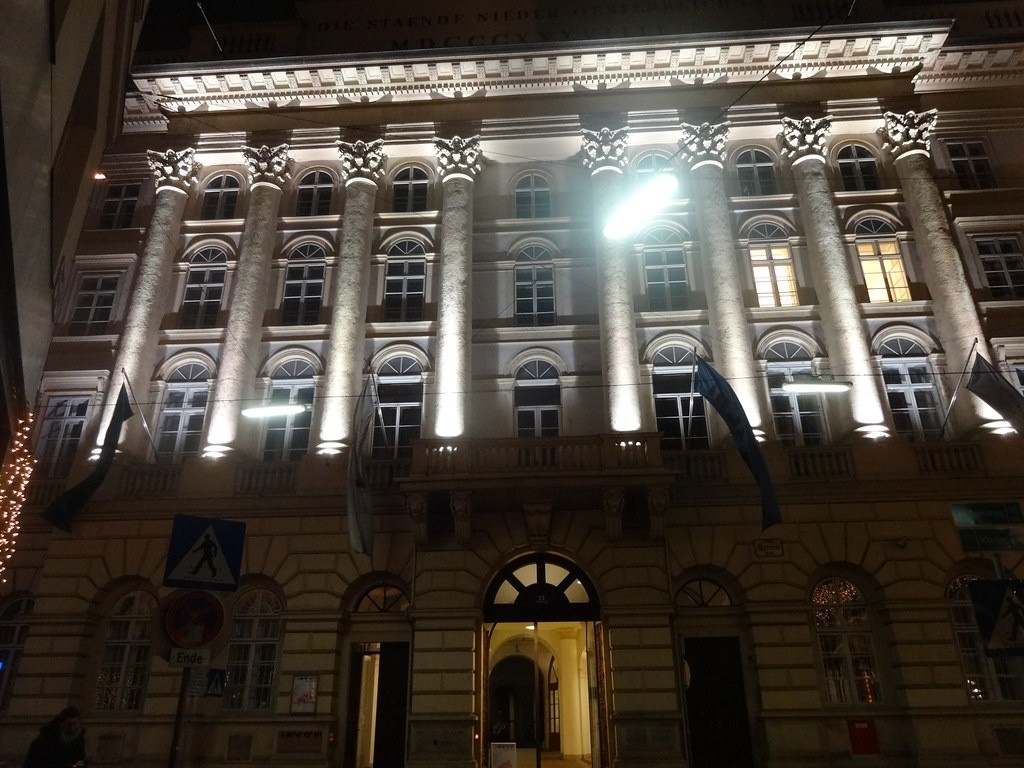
[965,351,1024,437]
[40,382,134,531]
[345,376,377,555]
[695,352,783,532]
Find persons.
[23,707,88,768]
